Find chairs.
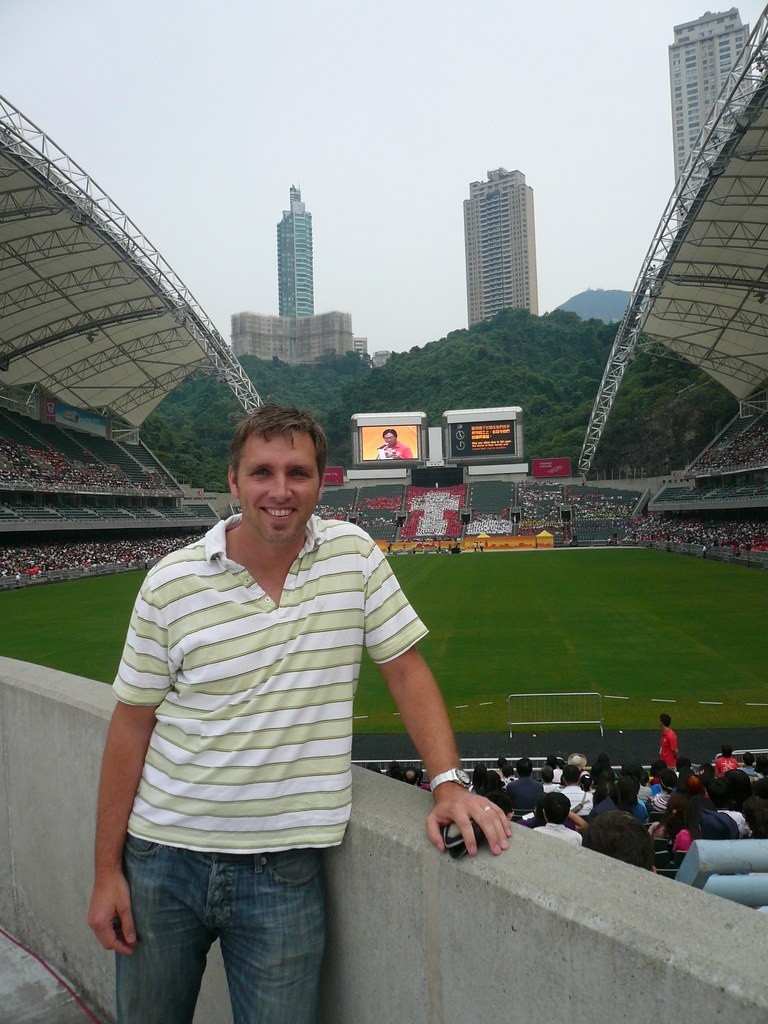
[513,809,687,879]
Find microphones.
[377,443,389,450]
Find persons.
[0,441,176,491]
[382,429,414,460]
[87,401,509,1024]
[0,533,205,586]
[359,745,768,879]
[658,713,679,769]
[622,513,768,559]
[685,424,768,472]
[312,478,637,554]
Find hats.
[568,753,587,771]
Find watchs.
[429,767,473,793]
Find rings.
[484,807,490,811]
